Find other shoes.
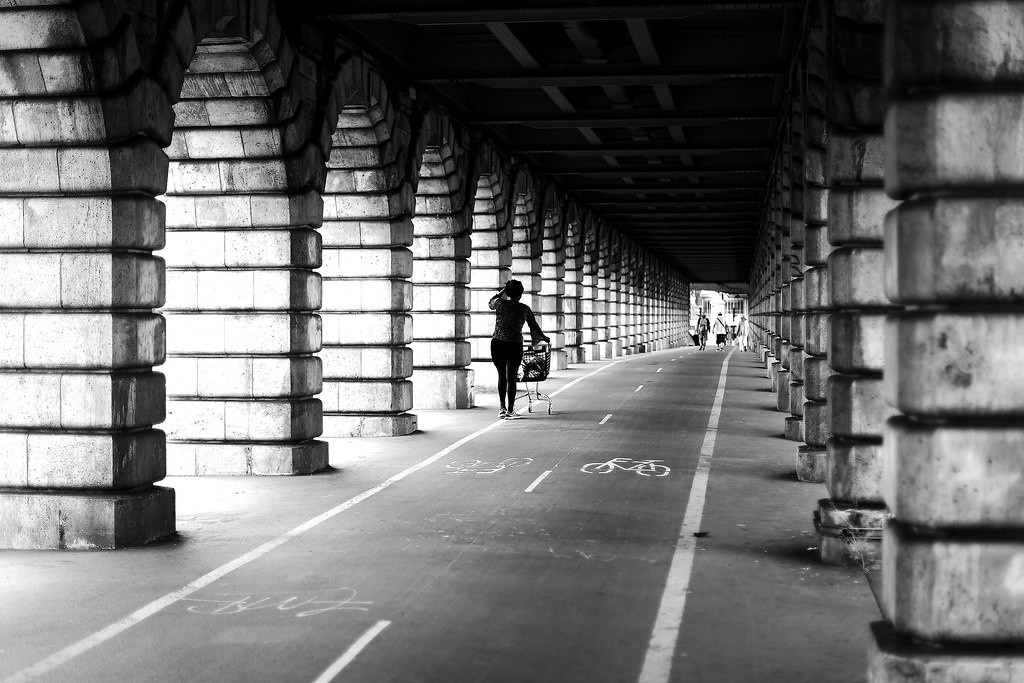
[744,345,748,352]
[698,346,706,350]
[740,350,744,353]
[715,348,724,352]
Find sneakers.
[498,409,523,420]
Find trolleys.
[513,339,554,416]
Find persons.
[713,312,728,353]
[696,315,711,350]
[488,280,552,421]
[737,316,749,352]
